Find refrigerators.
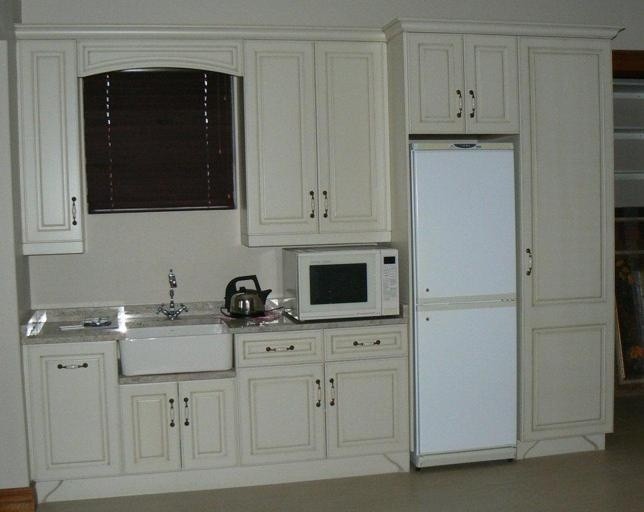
[410,134,522,469]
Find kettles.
[224,275,272,317]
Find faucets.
[167,267,178,313]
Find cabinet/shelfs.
[24,346,119,503]
[392,33,521,137]
[243,37,390,244]
[16,38,86,254]
[235,323,413,484]
[521,38,611,453]
[121,378,236,497]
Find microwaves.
[281,245,399,323]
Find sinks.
[119,323,235,376]
